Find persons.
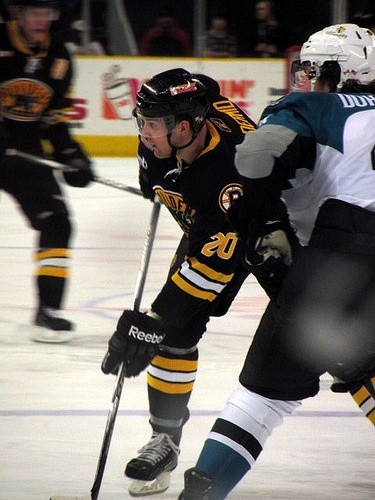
[179,22,375,500]
[101,67,375,497]
[197,15,237,58]
[254,2,282,59]
[139,15,194,56]
[0,0,95,345]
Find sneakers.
[33,311,72,344]
[123,434,183,497]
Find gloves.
[55,142,93,189]
[101,308,165,376]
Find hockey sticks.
[48,194,162,500]
[0,147,152,201]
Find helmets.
[298,23,375,94]
[134,67,210,151]
